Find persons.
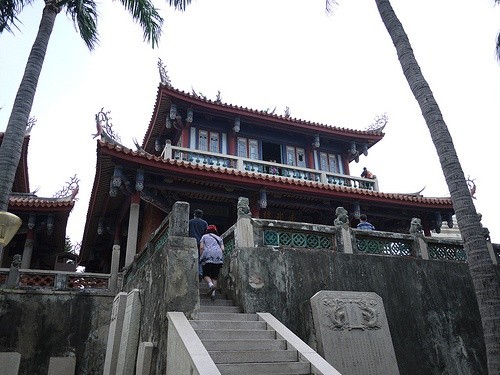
[360,166,367,177]
[200,224,225,300]
[357,214,375,231]
[189,209,209,281]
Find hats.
[206,225,218,232]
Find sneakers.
[210,291,216,298]
[207,284,214,294]
